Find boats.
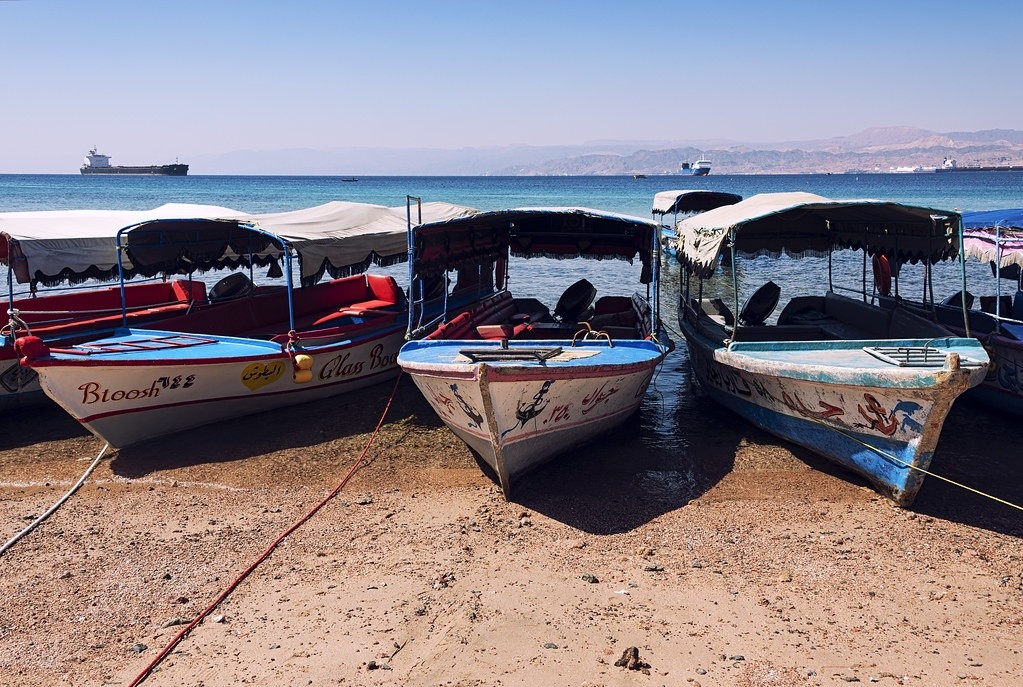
[682,154,712,179]
[396,195,678,502]
[16,201,486,449]
[865,208,1023,419]
[651,188,746,274]
[0,202,265,416]
[341,178,359,182]
[79,145,190,176]
[674,191,991,507]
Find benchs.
[431,292,638,342]
[0,273,400,338]
[694,291,959,342]
